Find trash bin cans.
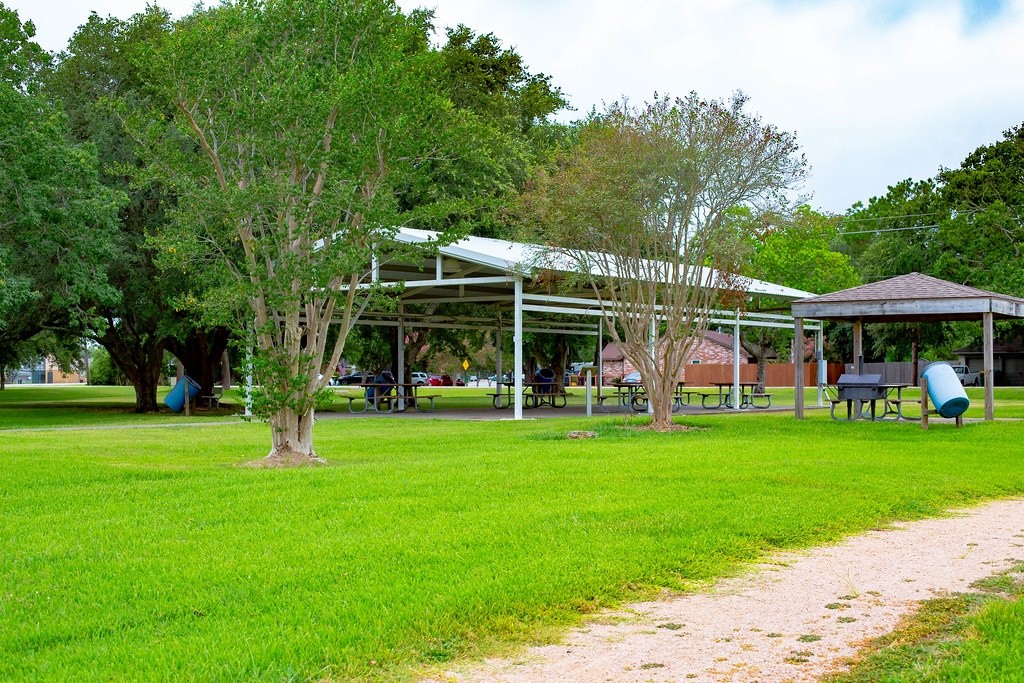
[164,374,202,413]
[532,368,556,395]
[920,360,971,419]
[364,371,396,406]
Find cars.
[469,376,478,381]
[488,374,509,381]
[382,371,395,380]
[427,374,453,386]
[319,374,335,386]
[392,371,427,386]
[531,368,577,386]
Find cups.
[623,378,641,384]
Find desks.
[709,382,759,408]
[674,381,696,405]
[497,382,559,407]
[613,384,643,411]
[360,384,422,413]
[879,385,909,418]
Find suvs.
[337,370,376,386]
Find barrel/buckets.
[920,361,970,418]
[164,375,201,413]
[364,373,395,405]
[532,368,555,394]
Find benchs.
[202,387,224,408]
[739,394,772,407]
[825,399,870,418]
[613,392,645,404]
[593,395,642,411]
[885,399,931,419]
[378,395,442,410]
[486,393,529,407]
[632,395,683,410]
[697,393,727,407]
[674,391,700,404]
[340,395,375,411]
[523,392,573,406]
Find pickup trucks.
[952,365,981,387]
